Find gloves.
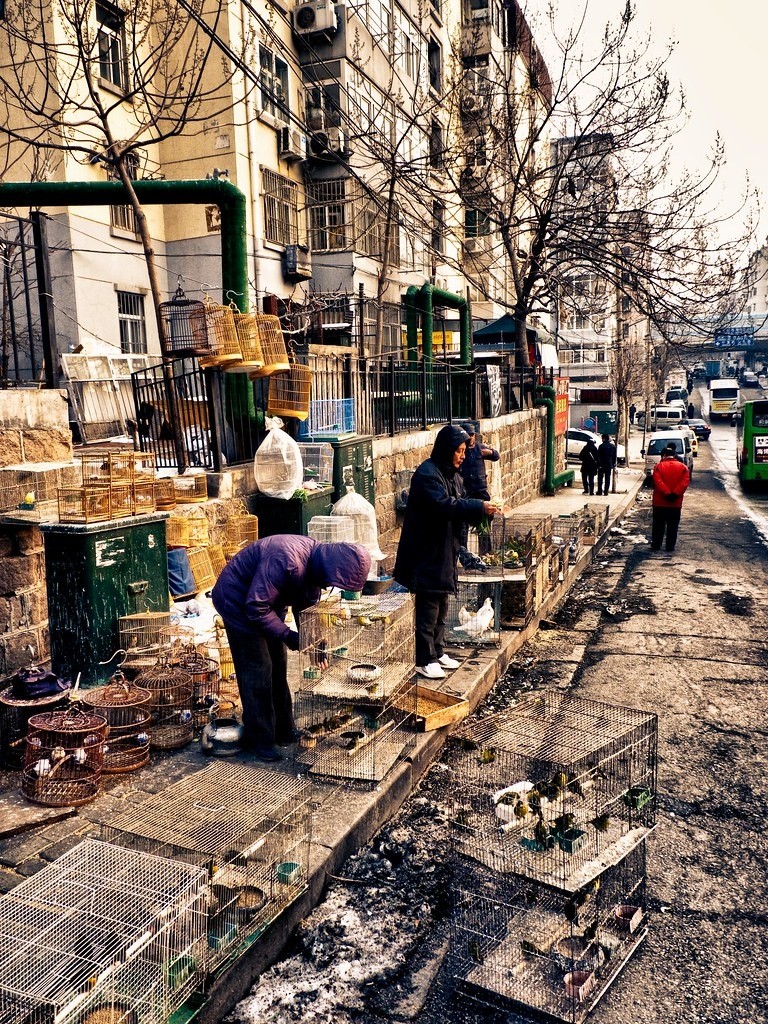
[666,493,678,502]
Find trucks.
[707,379,741,422]
[705,360,722,382]
[669,370,688,388]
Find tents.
[473,312,556,365]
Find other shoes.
[583,491,588,494]
[651,542,660,550]
[596,491,602,495]
[249,747,282,761]
[437,653,459,669]
[276,734,296,746]
[416,662,445,678]
[590,492,594,495]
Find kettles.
[200,700,243,756]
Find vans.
[640,431,693,483]
[637,384,689,429]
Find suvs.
[565,428,626,465]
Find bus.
[731,399,768,490]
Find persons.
[686,403,694,418]
[458,423,500,556]
[579,441,599,496]
[686,379,695,396]
[629,404,637,425]
[734,366,740,379]
[598,434,617,496]
[392,426,501,678]
[211,534,370,762]
[651,442,690,553]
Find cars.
[738,371,759,388]
[683,360,705,379]
[668,419,711,457]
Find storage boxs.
[391,681,470,733]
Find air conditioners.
[293,0,337,37]
[460,166,481,178]
[462,95,484,112]
[285,245,312,278]
[463,237,484,252]
[277,125,345,161]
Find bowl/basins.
[551,935,605,972]
[77,1003,139,1024]
[345,662,382,682]
[232,886,266,912]
[277,862,300,883]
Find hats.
[462,423,476,434]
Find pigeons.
[453,597,496,640]
[457,546,490,574]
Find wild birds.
[201,858,219,878]
[307,712,352,733]
[328,613,346,628]
[163,692,175,704]
[483,533,528,567]
[100,725,112,740]
[472,742,613,841]
[465,874,602,959]
[51,746,65,761]
[339,608,391,626]
[64,921,121,964]
[24,489,36,505]
[344,734,359,750]
